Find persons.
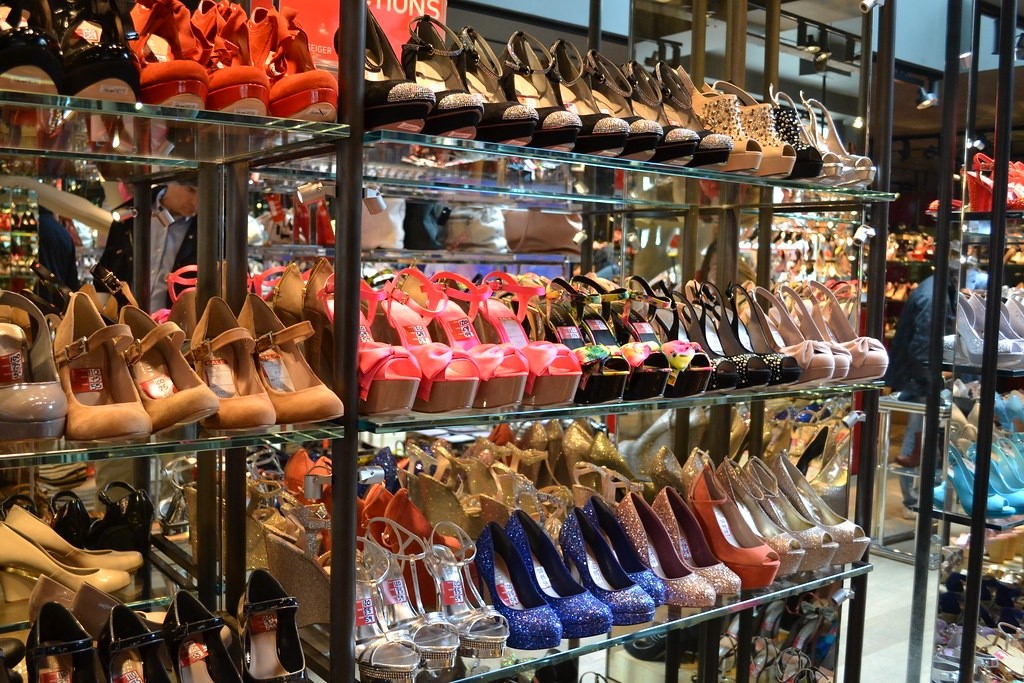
[594,248,618,284]
[93,184,199,513]
[700,242,716,292]
[38,204,82,311]
[890,239,984,520]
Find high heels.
[0,0,1024,683]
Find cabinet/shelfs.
[0,0,1024,683]
